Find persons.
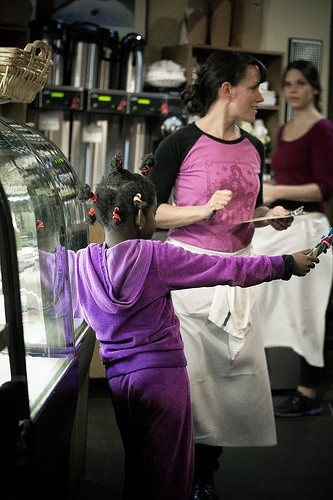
[78,152,320,500]
[147,50,294,500]
[263,59,333,417]
[9,193,78,380]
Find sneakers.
[274,390,323,416]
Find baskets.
[0,40,53,103]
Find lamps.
[57,0,134,31]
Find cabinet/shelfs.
[160,43,284,184]
[1,116,98,500]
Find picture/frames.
[118,0,148,42]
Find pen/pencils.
[206,210,217,224]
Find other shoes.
[194,484,212,500]
